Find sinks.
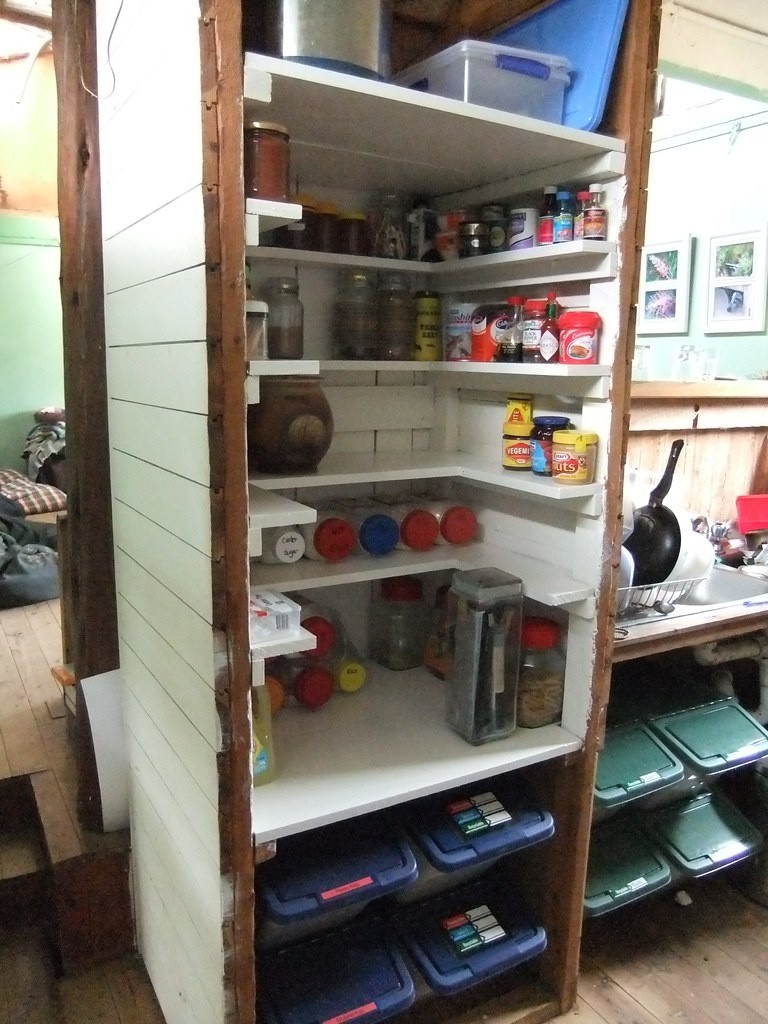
[679,567,768,604]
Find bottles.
[251,574,564,788]
[539,181,609,247]
[265,272,305,361]
[329,268,378,360]
[244,121,292,203]
[537,292,559,363]
[498,295,526,364]
[374,268,418,363]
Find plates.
[618,530,715,612]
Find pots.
[627,439,684,589]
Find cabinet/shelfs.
[76,0,666,1024]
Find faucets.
[692,515,712,539]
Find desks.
[28,509,67,546]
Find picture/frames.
[636,233,691,335]
[704,229,767,334]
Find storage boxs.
[593,715,685,825]
[386,870,546,997]
[390,40,571,125]
[584,814,671,922]
[394,788,557,905]
[635,791,764,894]
[632,692,768,811]
[254,818,419,951]
[254,905,417,1024]
